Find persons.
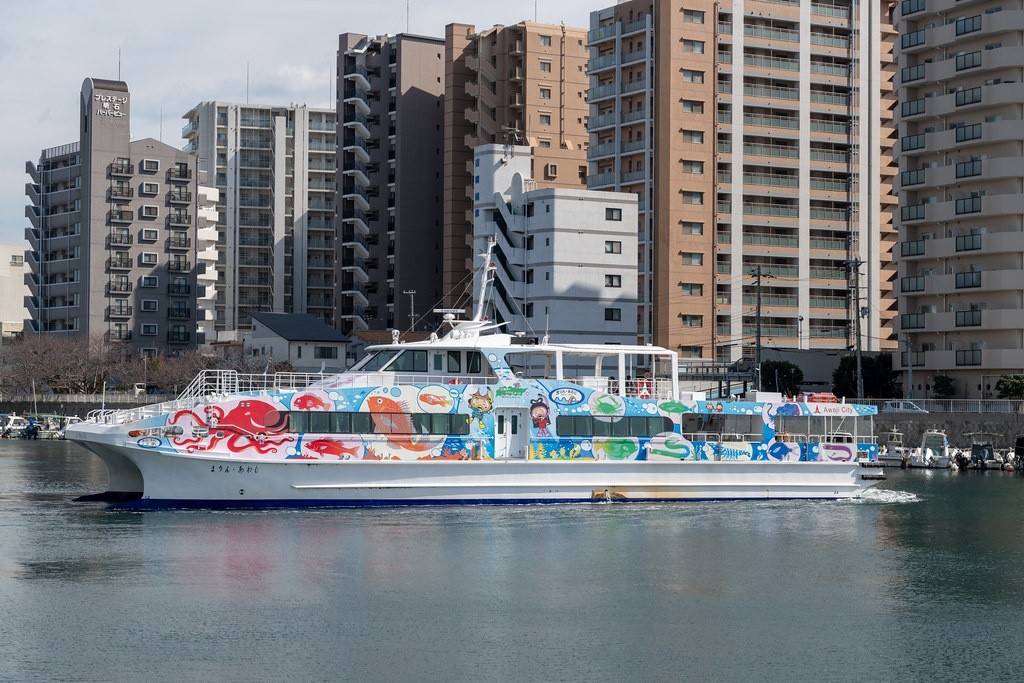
[608,376,619,394]
[625,375,635,398]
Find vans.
[881,399,929,413]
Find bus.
[102,381,147,396]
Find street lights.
[402,285,417,331]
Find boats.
[65,232,885,509]
[0,410,86,440]
[878,425,1009,470]
